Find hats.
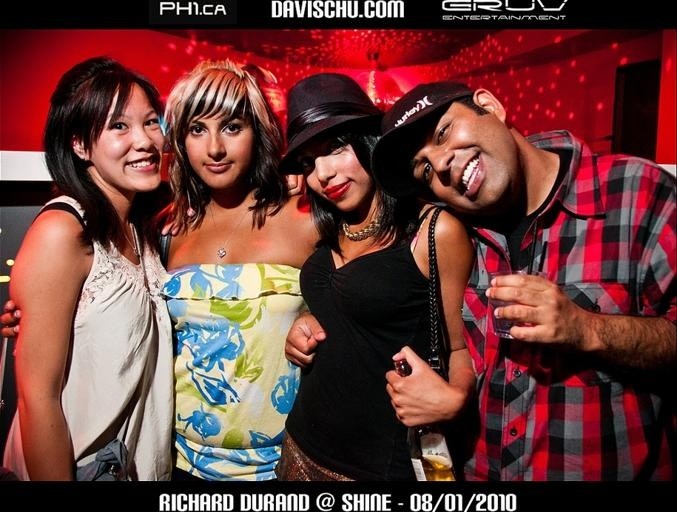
[371,80,474,201]
[272,72,383,175]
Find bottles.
[394,359,462,481]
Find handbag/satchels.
[384,366,481,481]
[77,440,139,485]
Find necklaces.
[209,202,248,258]
[339,217,380,241]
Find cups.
[488,272,531,342]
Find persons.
[372,80,677,482]
[276,73,476,482]
